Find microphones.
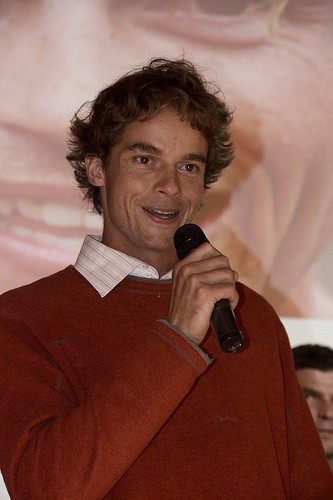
[174,222,244,355]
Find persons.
[0,47,333,500]
[289,345,332,467]
